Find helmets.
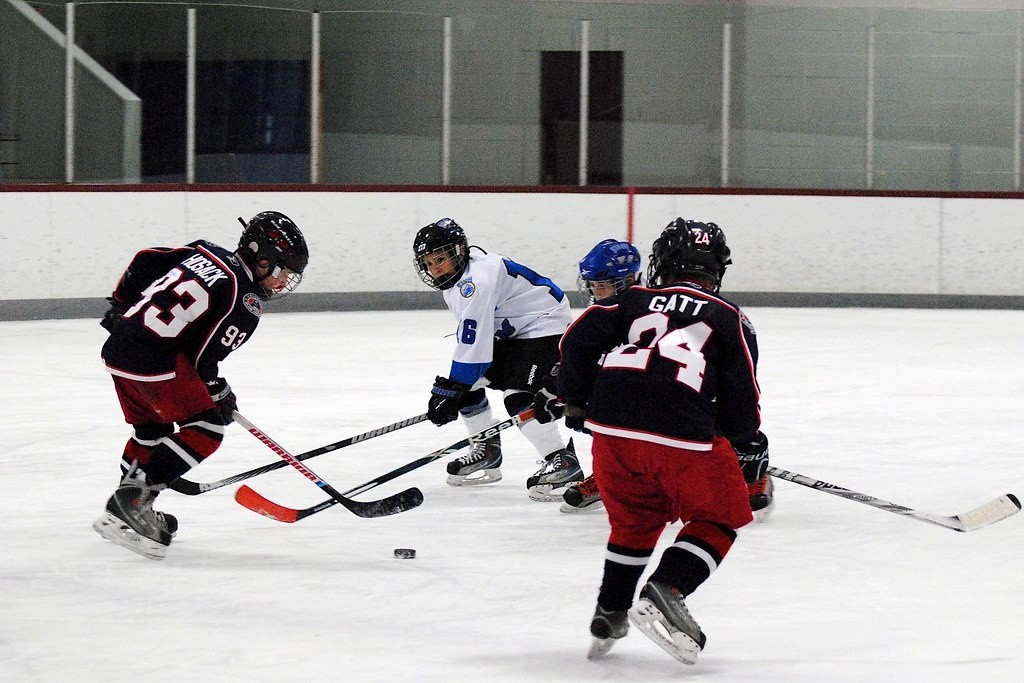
[412,218,470,291]
[575,239,641,306]
[238,211,309,302]
[646,217,730,294]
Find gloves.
[100,291,142,335]
[732,431,772,511]
[530,361,567,425]
[207,376,238,423]
[558,364,602,435]
[427,374,472,428]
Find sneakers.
[559,474,604,514]
[627,580,706,664]
[101,476,178,538]
[91,456,171,561]
[587,605,628,658]
[527,437,584,501]
[446,431,502,486]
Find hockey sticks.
[765,463,1023,534]
[235,398,567,524]
[165,412,431,497]
[232,410,425,518]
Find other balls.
[393,548,417,560]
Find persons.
[559,217,772,667]
[91,210,309,560]
[560,238,648,513]
[412,218,584,502]
